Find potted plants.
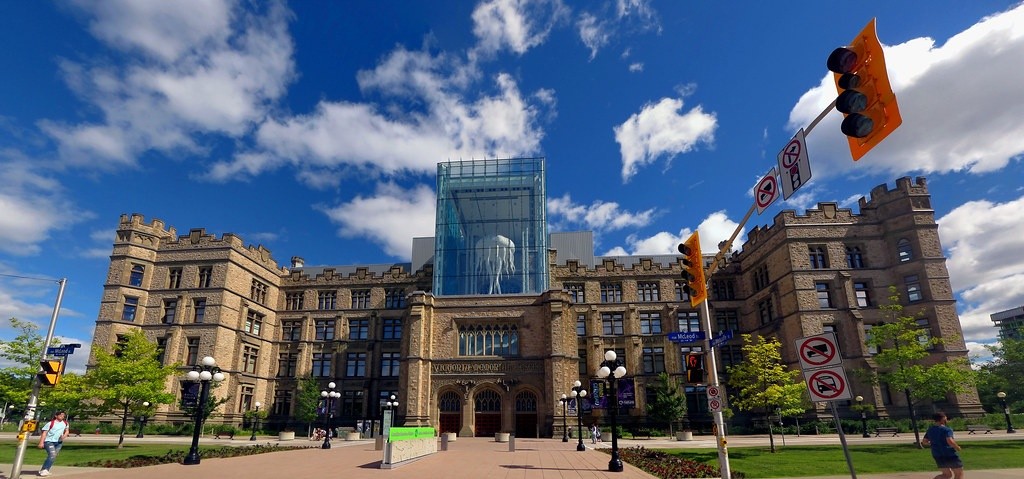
[495,431,511,442]
[601,429,612,442]
[346,430,360,440]
[279,428,295,440]
[443,431,457,441]
[676,429,692,440]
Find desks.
[872,427,899,437]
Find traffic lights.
[39,354,62,386]
[686,353,705,384]
[677,230,709,306]
[825,16,901,161]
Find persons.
[310,427,323,441]
[923,412,965,479]
[590,424,601,444]
[38,411,70,476]
[328,428,333,440]
[96,427,100,435]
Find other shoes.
[39,469,49,476]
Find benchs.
[64,429,81,437]
[967,424,992,434]
[214,430,234,439]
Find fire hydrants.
[95,428,100,435]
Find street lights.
[595,350,628,473]
[996,391,1016,433]
[387,395,399,427]
[569,380,588,452]
[31,401,47,437]
[320,382,341,449]
[249,402,261,441]
[183,357,226,465]
[135,401,152,439]
[855,396,871,438]
[559,394,569,442]
